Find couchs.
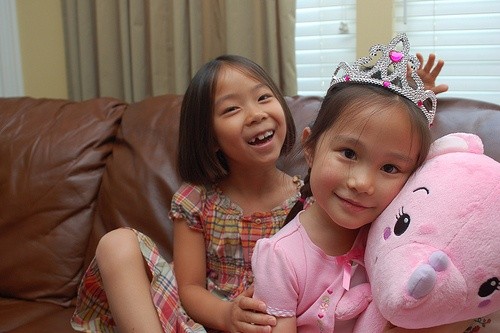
[0,96,500,333]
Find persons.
[250,33,437,333]
[71,53,448,333]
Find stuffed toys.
[334,133,500,333]
